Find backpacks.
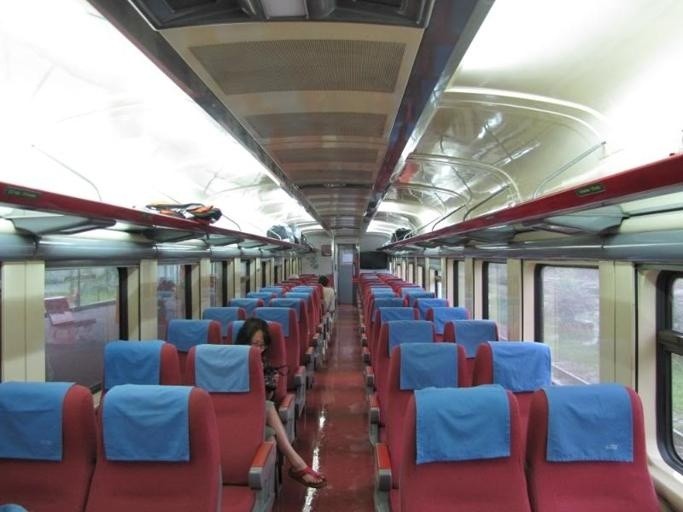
[142,203,222,243]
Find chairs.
[82,384,224,510]
[100,338,182,400]
[201,270,337,417]
[367,318,437,447]
[371,341,473,511]
[395,382,531,511]
[443,318,499,373]
[527,380,664,512]
[164,319,226,384]
[477,338,553,450]
[354,269,473,396]
[1,378,98,512]
[186,342,280,510]
[225,318,297,445]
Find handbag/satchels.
[392,228,412,242]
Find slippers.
[290,467,327,488]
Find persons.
[233,317,328,489]
[318,275,336,314]
